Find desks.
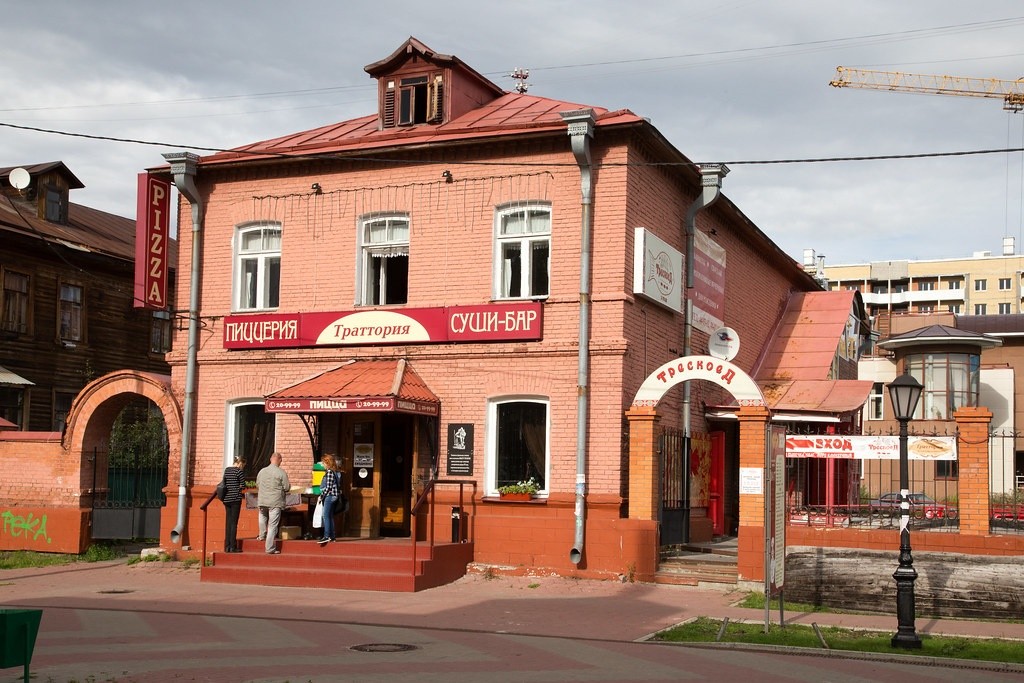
[299,492,323,540]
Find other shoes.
[317,537,332,543]
[257,536,263,541]
[225,546,230,552]
[274,550,281,554]
[230,548,242,552]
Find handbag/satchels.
[334,495,346,515]
[217,478,227,501]
[312,498,324,528]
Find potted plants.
[497,476,541,501]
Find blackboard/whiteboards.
[446,423,474,476]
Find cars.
[867,493,939,514]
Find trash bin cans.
[452,507,460,544]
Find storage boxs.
[279,526,302,542]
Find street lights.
[885,365,927,651]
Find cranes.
[829,65,1024,111]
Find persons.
[223,455,247,553]
[256,453,290,554]
[334,456,349,513]
[317,454,340,544]
